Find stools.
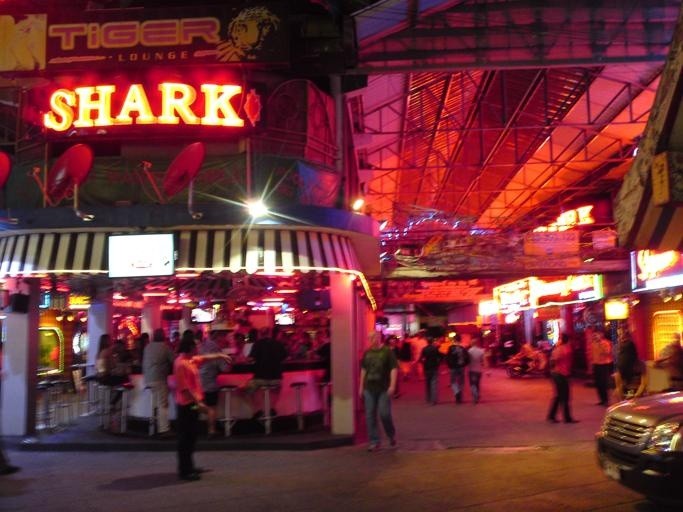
[32,376,331,438]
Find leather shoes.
[179,472,199,480]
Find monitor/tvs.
[190,307,215,323]
[106,232,174,278]
[274,313,295,325]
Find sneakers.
[388,440,399,449]
[369,441,380,451]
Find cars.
[594,390,683,508]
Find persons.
[660,332,683,391]
[184,327,332,422]
[174,337,232,477]
[588,328,648,406]
[547,333,579,425]
[358,331,400,451]
[0,342,22,476]
[95,329,194,438]
[387,333,487,403]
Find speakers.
[3,293,28,314]
